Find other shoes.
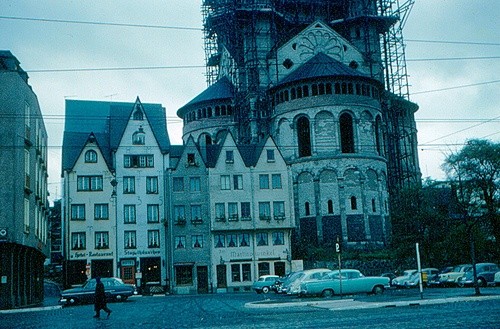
[93,315,100,318]
[107,309,113,318]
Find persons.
[93,275,112,318]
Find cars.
[58,278,135,306]
[252,262,500,298]
